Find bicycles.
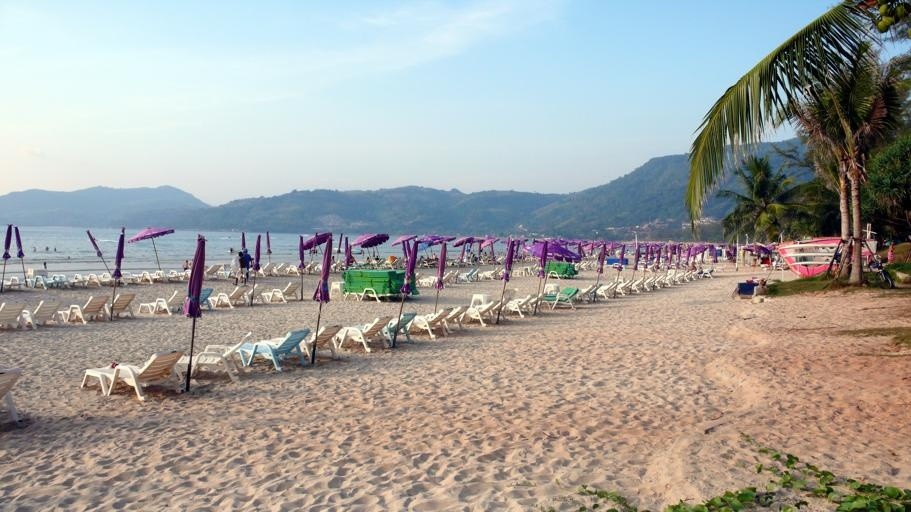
[872,257,893,289]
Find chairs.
[2,268,191,330]
[235,329,313,371]
[328,259,418,304]
[261,325,343,364]
[405,295,501,343]
[175,332,254,383]
[81,349,185,403]
[333,315,390,354]
[492,286,601,321]
[355,312,416,349]
[503,251,600,280]
[1,368,22,427]
[200,262,321,312]
[601,252,714,300]
[415,252,503,287]
